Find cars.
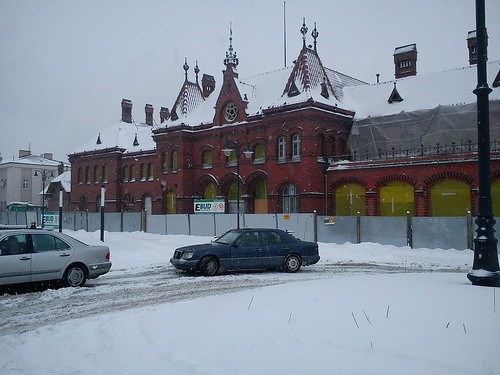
[169,228,320,276]
[0,228,111,287]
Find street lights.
[34,169,54,229]
